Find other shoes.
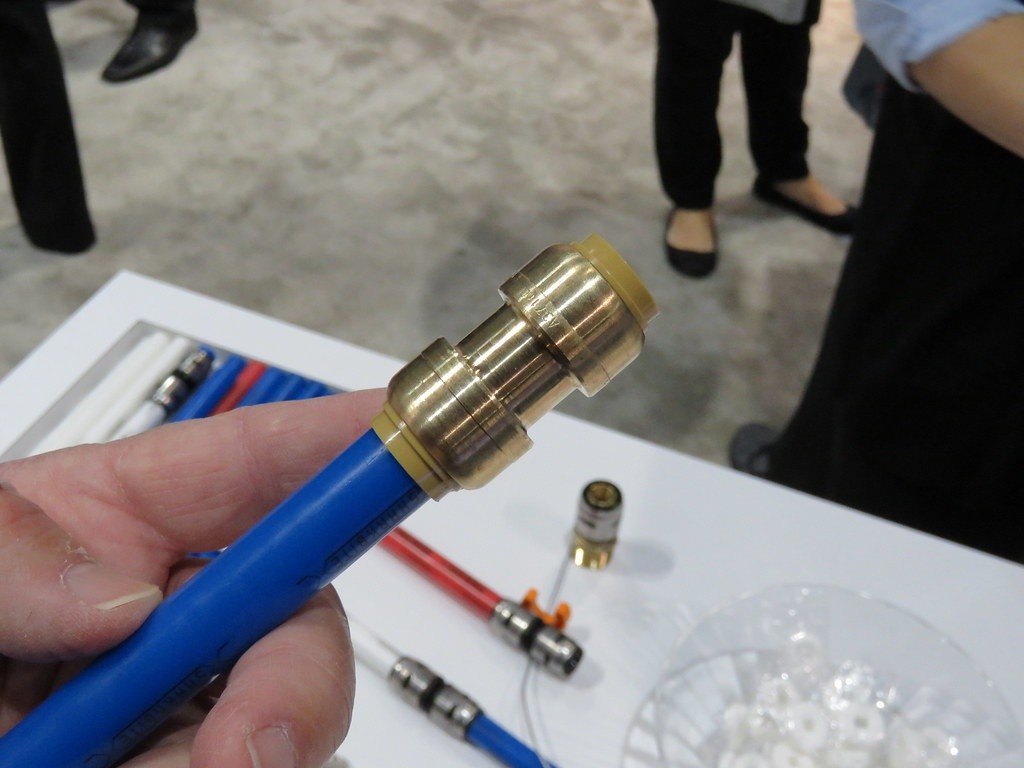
[729,423,786,485]
[664,204,718,277]
[752,172,860,235]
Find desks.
[0,270,1024,768]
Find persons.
[651,1,859,278]
[759,1,1024,576]
[0,388,390,768]
[100,0,197,83]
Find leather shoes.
[103,12,196,83]
